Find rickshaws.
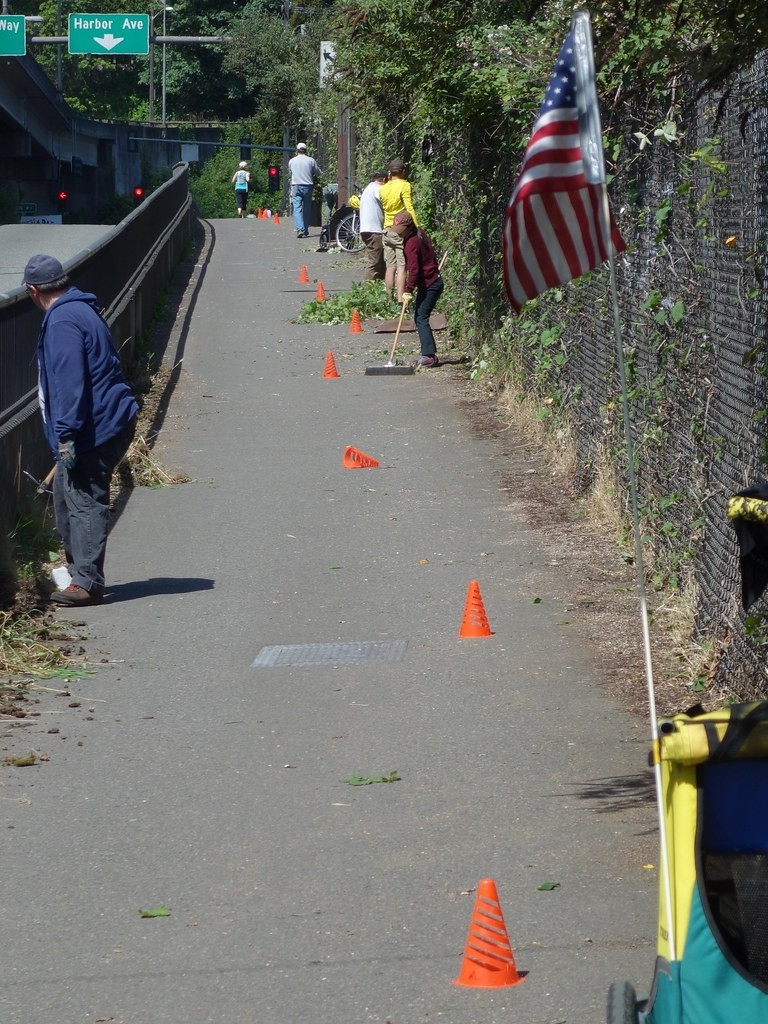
[320,196,356,251]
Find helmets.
[349,195,360,210]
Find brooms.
[365,301,414,376]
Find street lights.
[147,5,174,121]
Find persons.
[360,162,418,314]
[232,161,252,218]
[289,143,321,238]
[20,255,139,606]
[390,210,444,367]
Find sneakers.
[50,584,103,606]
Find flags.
[502,22,629,315]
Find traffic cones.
[258,208,268,219]
[450,878,526,989]
[273,213,280,224]
[343,446,380,468]
[349,309,363,332]
[459,580,494,638]
[299,265,309,282]
[323,352,338,377]
[316,282,325,300]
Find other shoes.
[297,231,304,238]
[413,356,438,367]
[304,232,309,236]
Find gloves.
[58,439,76,470]
[402,293,413,308]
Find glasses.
[25,287,30,294]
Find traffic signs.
[68,12,149,55]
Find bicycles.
[335,182,370,252]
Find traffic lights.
[58,191,70,211]
[268,167,280,190]
[134,187,145,209]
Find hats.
[21,255,65,286]
[297,143,306,149]
[390,212,412,234]
[389,160,406,172]
[239,162,247,167]
